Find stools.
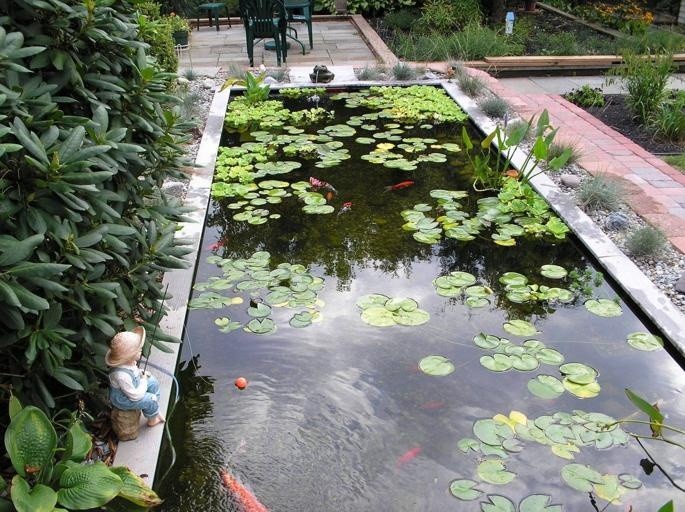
[197,3,232,31]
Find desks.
[281,1,313,49]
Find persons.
[104,325,165,428]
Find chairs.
[238,0,287,68]
